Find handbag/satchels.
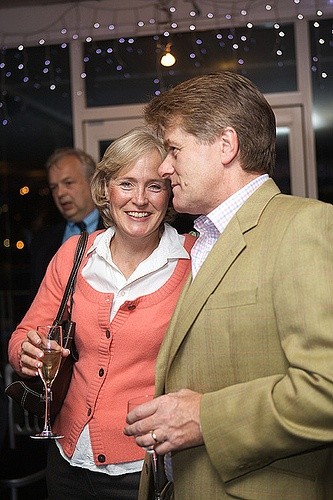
[6,232,89,420]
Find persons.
[123,72,333,500]
[8,126,199,500]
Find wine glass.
[126,395,170,500]
[29,326,64,439]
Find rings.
[152,430,159,444]
